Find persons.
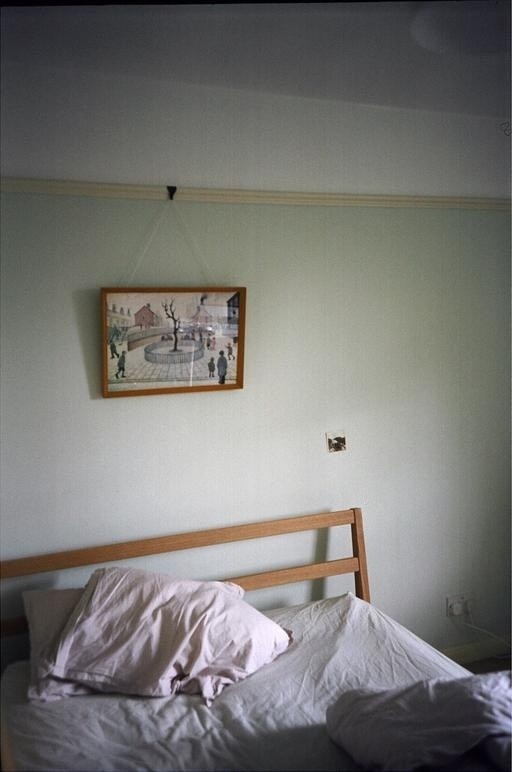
[211,337,216,350]
[216,350,228,384]
[206,333,211,350]
[208,356,216,377]
[224,342,235,360]
[115,351,126,379]
[109,339,119,359]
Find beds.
[0,507,510,771]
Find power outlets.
[444,592,475,621]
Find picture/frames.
[97,284,248,399]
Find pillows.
[36,566,292,704]
[21,581,245,704]
